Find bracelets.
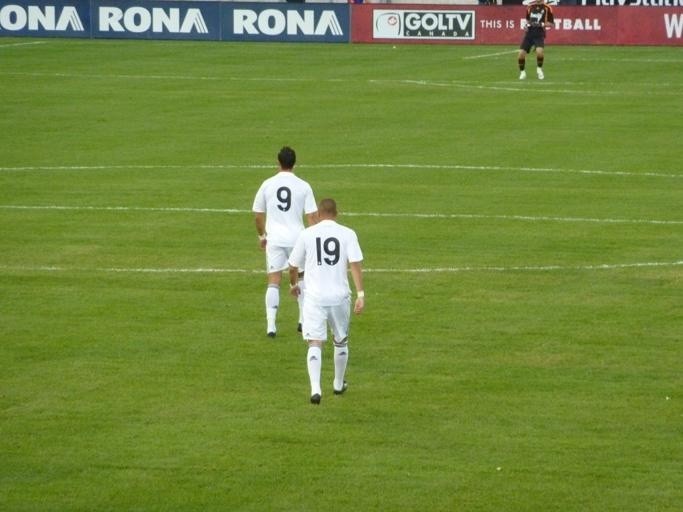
[356,290,364,298]
[257,233,267,240]
[289,282,298,290]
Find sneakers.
[334,380,347,394]
[519,70,526,80]
[311,393,320,405]
[536,66,544,80]
[267,327,276,337]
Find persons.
[247,145,318,338]
[516,0,555,83]
[287,198,366,406]
